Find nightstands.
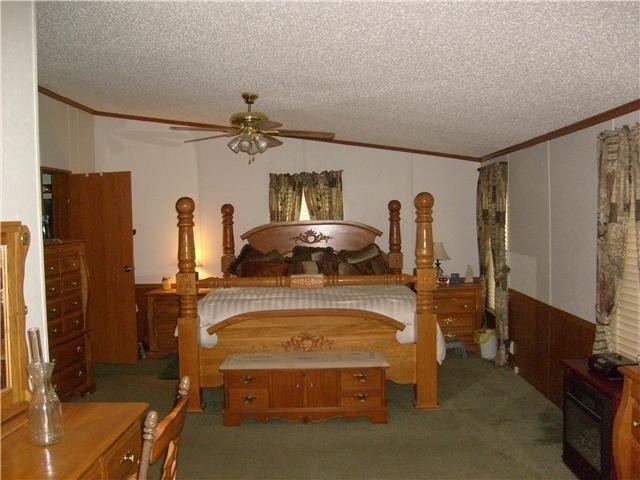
[145,284,209,359]
[431,277,485,353]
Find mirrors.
[0,223,36,422]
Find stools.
[446,339,468,360]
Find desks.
[1,402,150,480]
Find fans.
[170,93,336,159]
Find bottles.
[464,266,474,284]
[25,360,65,446]
[162,275,171,290]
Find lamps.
[430,242,449,274]
[224,132,277,154]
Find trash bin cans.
[475,329,498,360]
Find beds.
[172,192,444,426]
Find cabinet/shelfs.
[556,355,622,479]
[613,363,640,480]
[219,350,390,424]
[44,237,98,403]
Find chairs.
[131,376,192,479]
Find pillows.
[225,242,396,277]
